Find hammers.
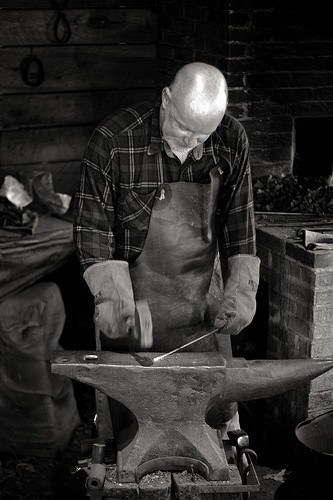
[96,302,153,348]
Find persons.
[72,61,261,479]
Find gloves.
[215,254,261,336]
[83,260,136,339]
